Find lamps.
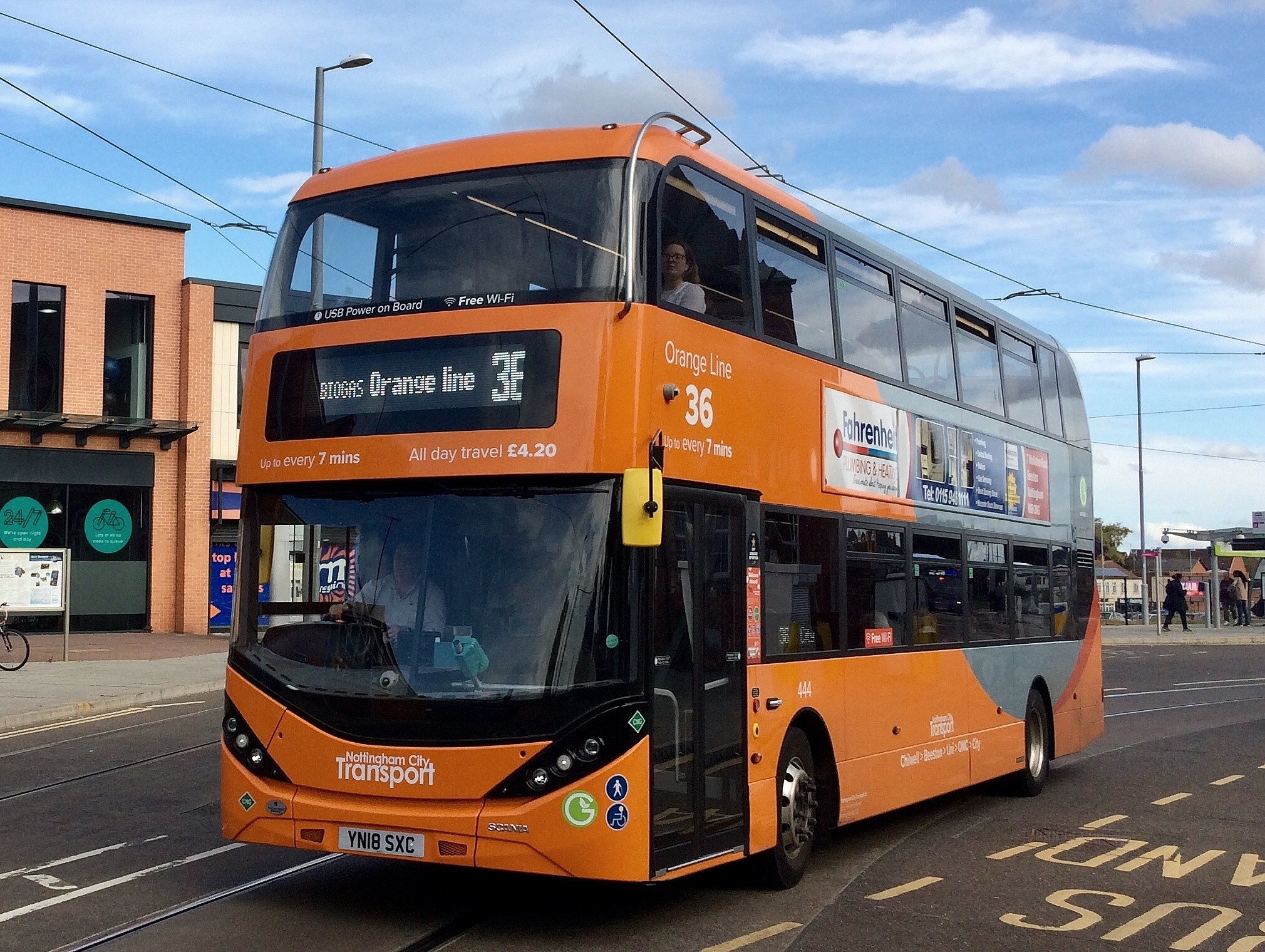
[44,499,64,513]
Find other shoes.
[1232,623,1244,627]
[1234,618,1238,622]
[1242,623,1251,627]
[1223,621,1230,625]
[1162,627,1171,632]
[1183,629,1192,632]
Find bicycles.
[0,602,30,671]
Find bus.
[216,110,1103,891]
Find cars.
[1100,611,1124,621]
[1120,609,1168,621]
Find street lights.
[1190,549,1196,576]
[1136,355,1157,624]
[301,55,372,621]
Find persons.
[1228,570,1250,627]
[329,539,446,657]
[659,239,708,317]
[1161,573,1192,632]
[1220,572,1239,626]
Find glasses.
[661,252,687,263]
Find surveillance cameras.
[1161,536,1169,544]
